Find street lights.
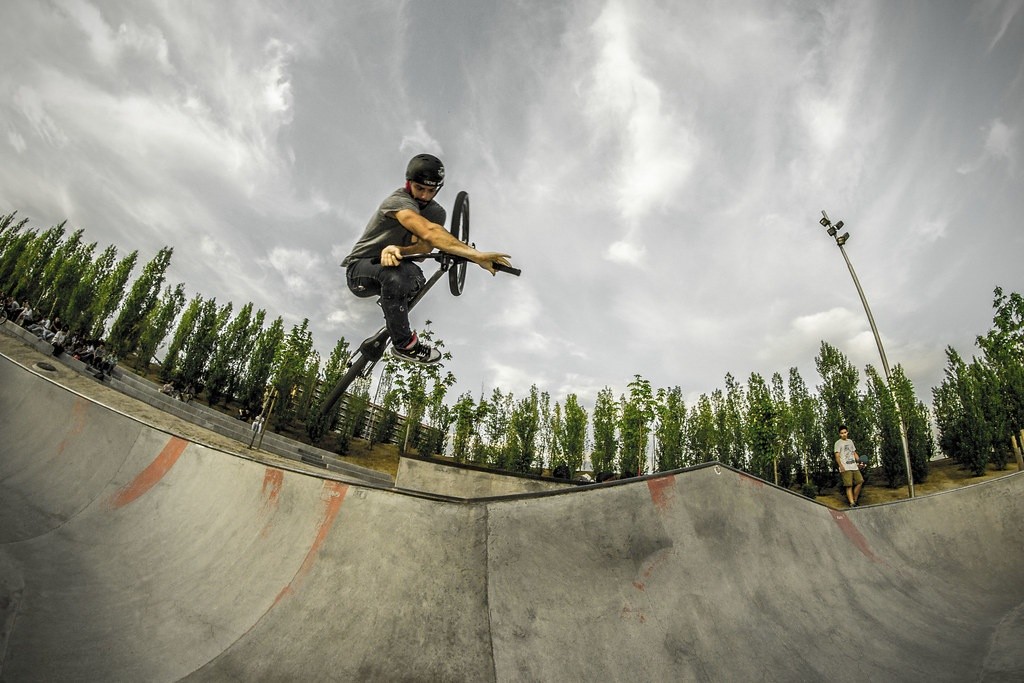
[819,209,915,499]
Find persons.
[252,413,264,432]
[157,379,174,397]
[339,154,513,366]
[180,382,196,404]
[833,425,864,508]
[239,409,250,421]
[0,292,117,374]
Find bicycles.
[0,297,9,325]
[318,190,522,417]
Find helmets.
[406,154,445,187]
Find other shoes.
[850,504,860,507]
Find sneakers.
[391,331,441,365]
